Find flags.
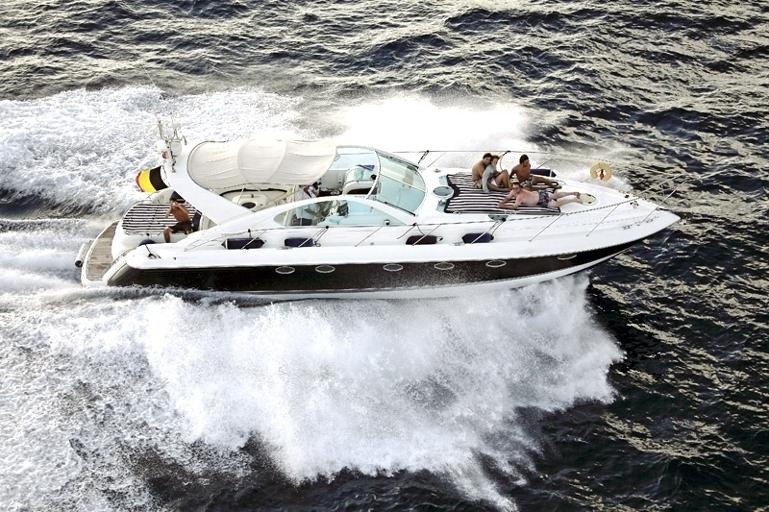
[136,166,168,192]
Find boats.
[73,114,694,295]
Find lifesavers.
[590,162,612,183]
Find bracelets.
[314,188,320,194]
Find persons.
[472,152,511,191]
[498,182,582,209]
[163,198,192,242]
[295,182,324,225]
[482,156,509,192]
[508,154,563,191]
